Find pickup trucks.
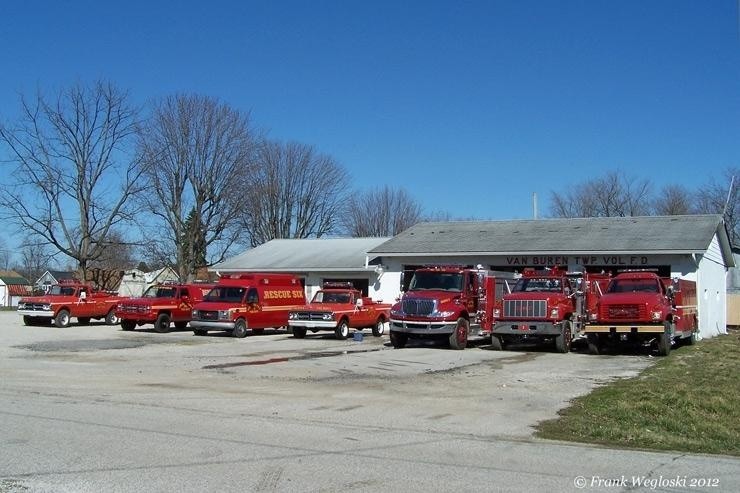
[17,280,129,327]
[287,281,392,339]
[115,284,221,333]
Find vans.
[191,272,307,337]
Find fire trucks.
[583,268,700,353]
[494,268,586,354]
[390,264,523,355]
